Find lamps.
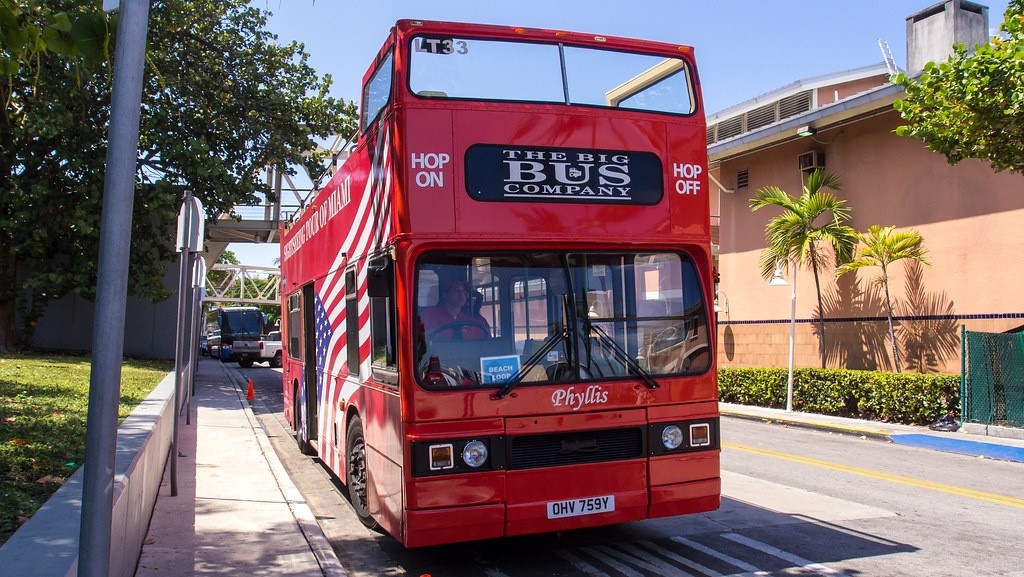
[797,126,816,137]
[713,290,729,314]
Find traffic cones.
[245,380,256,400]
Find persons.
[421,280,491,339]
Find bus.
[275,19,721,549]
[218,306,268,364]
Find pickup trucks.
[232,331,283,369]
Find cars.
[205,328,221,347]
